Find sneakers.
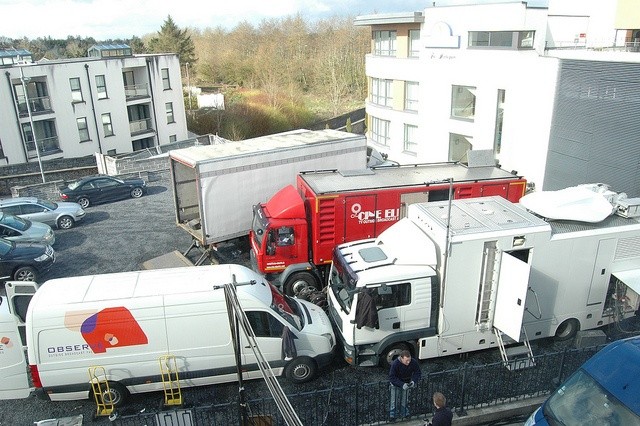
[403,413,413,418]
[388,417,396,421]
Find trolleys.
[88,367,119,423]
[159,356,187,411]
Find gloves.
[410,381,414,389]
[402,382,409,391]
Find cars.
[1,236,57,283]
[0,196,87,229]
[1,211,55,247]
[58,174,147,209]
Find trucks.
[0,263,336,409]
[523,335,638,425]
[169,128,398,246]
[248,148,527,298]
[326,182,640,373]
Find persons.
[387,349,420,422]
[423,392,453,426]
